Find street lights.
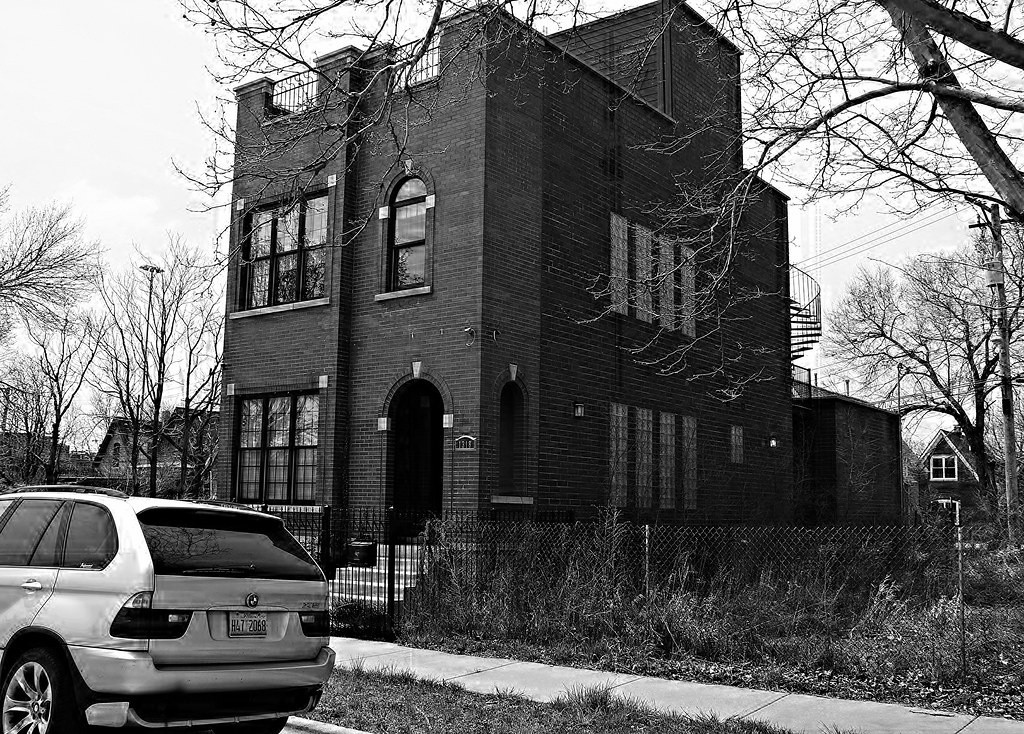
[139,263,164,426]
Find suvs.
[1,484,336,734]
